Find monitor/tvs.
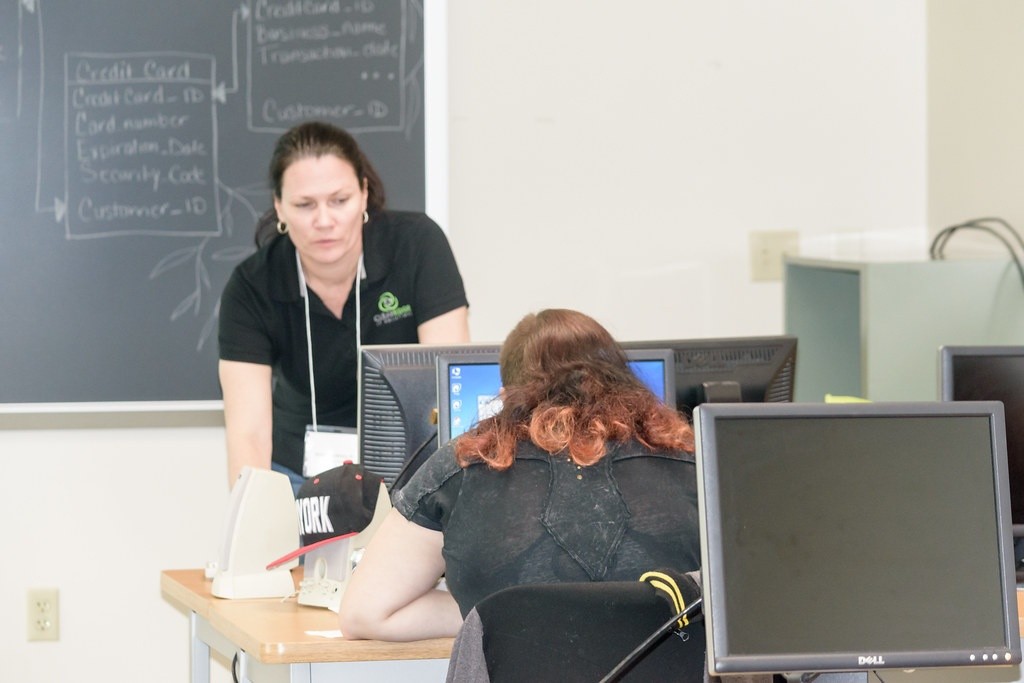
[356,344,507,494]
[692,401,1022,683]
[935,344,1024,538]
[614,334,798,427]
[436,349,676,452]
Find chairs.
[444,570,775,683]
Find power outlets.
[26,587,61,642]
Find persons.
[337,309,702,643]
[214,120,472,566]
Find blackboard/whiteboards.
[0,0,446,414]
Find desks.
[156,565,456,683]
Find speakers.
[297,482,391,612]
[210,469,295,597]
